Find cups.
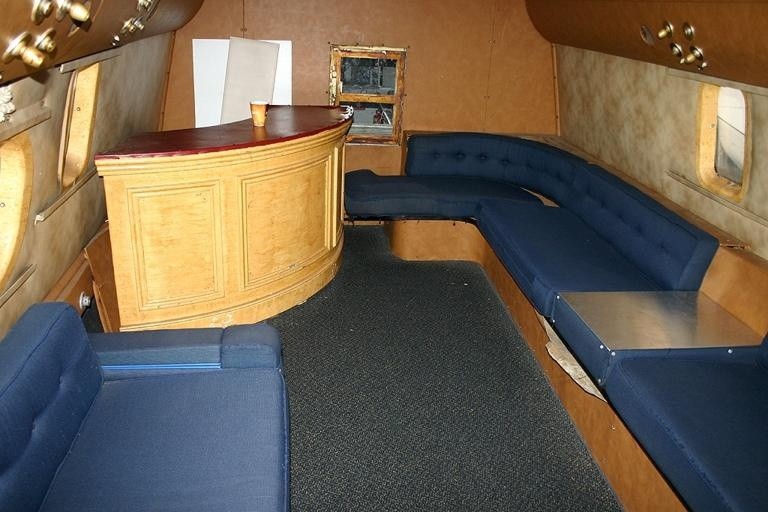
[248,100,270,128]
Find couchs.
[1,301,295,511]
